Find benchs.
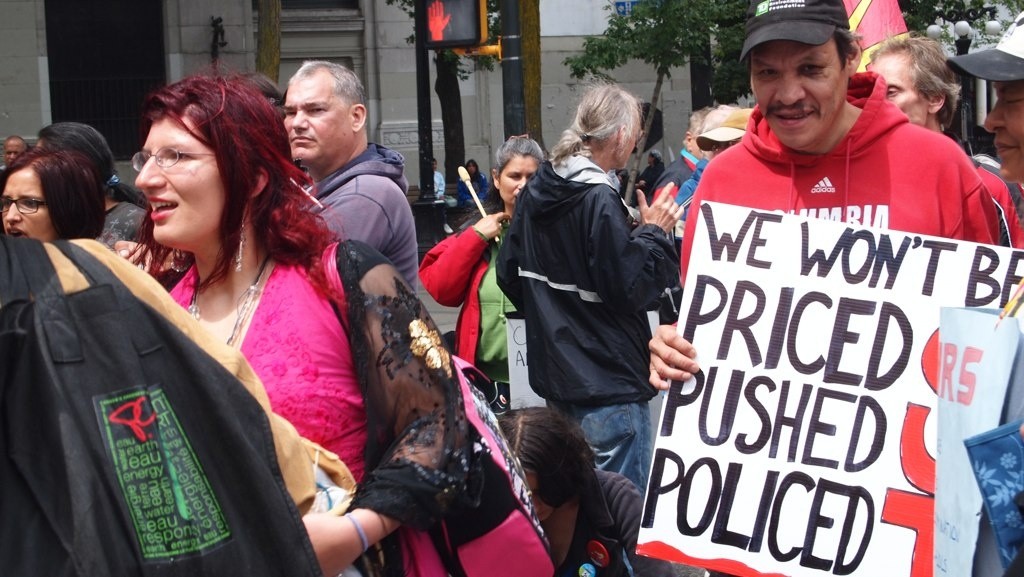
[405,184,459,205]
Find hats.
[739,0,851,65]
[946,11,1024,82]
[652,150,661,161]
[696,108,754,151]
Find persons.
[418,10,1024,577]
[648,0,1001,577]
[0,59,417,294]
[133,72,469,577]
[1,232,322,577]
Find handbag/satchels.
[964,417,1024,571]
[321,239,556,577]
[0,238,325,577]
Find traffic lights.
[421,0,481,48]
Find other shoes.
[444,224,454,234]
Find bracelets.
[344,513,369,553]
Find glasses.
[131,147,216,171]
[711,141,741,149]
[0,197,47,214]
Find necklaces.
[188,253,270,348]
